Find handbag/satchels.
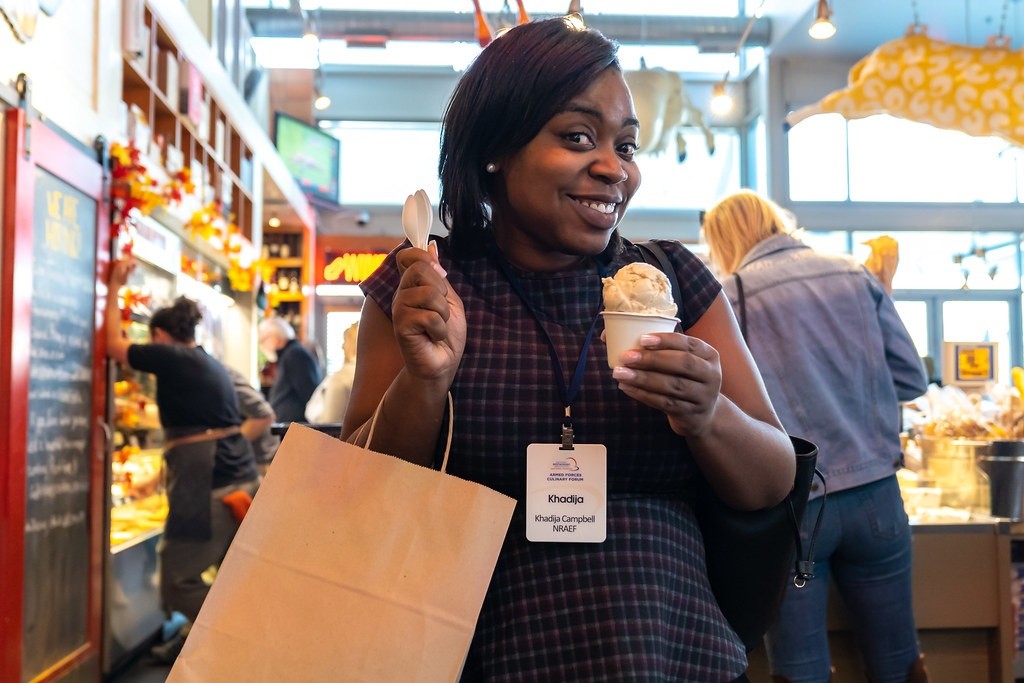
[637,239,819,651]
[157,368,520,683]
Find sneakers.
[150,611,192,662]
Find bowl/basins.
[919,436,991,509]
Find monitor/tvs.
[272,111,340,207]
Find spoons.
[402,189,433,250]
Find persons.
[256,316,320,424]
[702,188,931,683]
[105,258,261,659]
[220,362,281,473]
[340,13,798,681]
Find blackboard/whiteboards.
[1,106,115,683]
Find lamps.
[807,1,837,41]
[563,1,586,32]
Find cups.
[599,311,681,369]
[988,440,1024,518]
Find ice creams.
[598,261,683,371]
